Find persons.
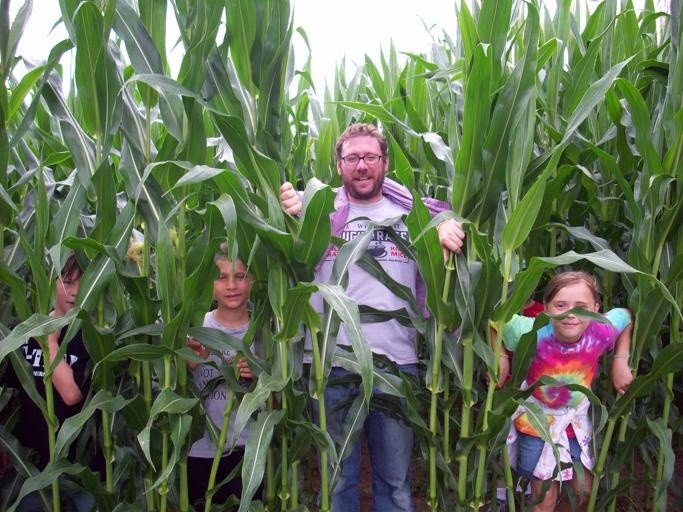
[178,244,273,511]
[488,270,634,511]
[275,119,467,511]
[0,250,112,511]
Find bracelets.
[611,353,628,362]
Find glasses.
[341,153,384,166]
